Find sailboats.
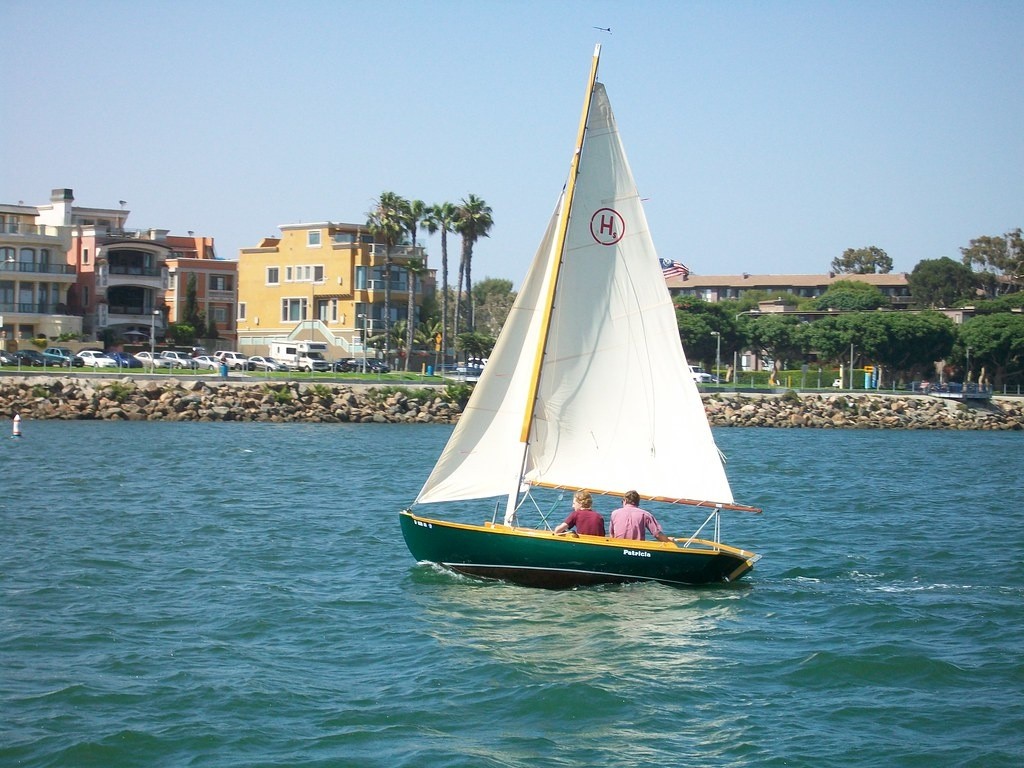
[394,35,764,595]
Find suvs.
[687,365,712,383]
[40,346,85,367]
[214,350,255,371]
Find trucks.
[269,340,332,372]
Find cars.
[193,354,230,371]
[353,357,391,373]
[133,351,173,367]
[0,350,18,366]
[161,350,200,370]
[76,350,118,368]
[12,350,53,367]
[248,355,289,373]
[333,357,357,373]
[107,351,144,369]
[467,357,488,370]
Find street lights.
[150,309,162,374]
[358,313,368,376]
[709,331,721,388]
[966,346,974,375]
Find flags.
[659,258,690,282]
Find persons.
[609,491,675,544]
[220,358,229,381]
[554,492,605,537]
[919,378,949,394]
